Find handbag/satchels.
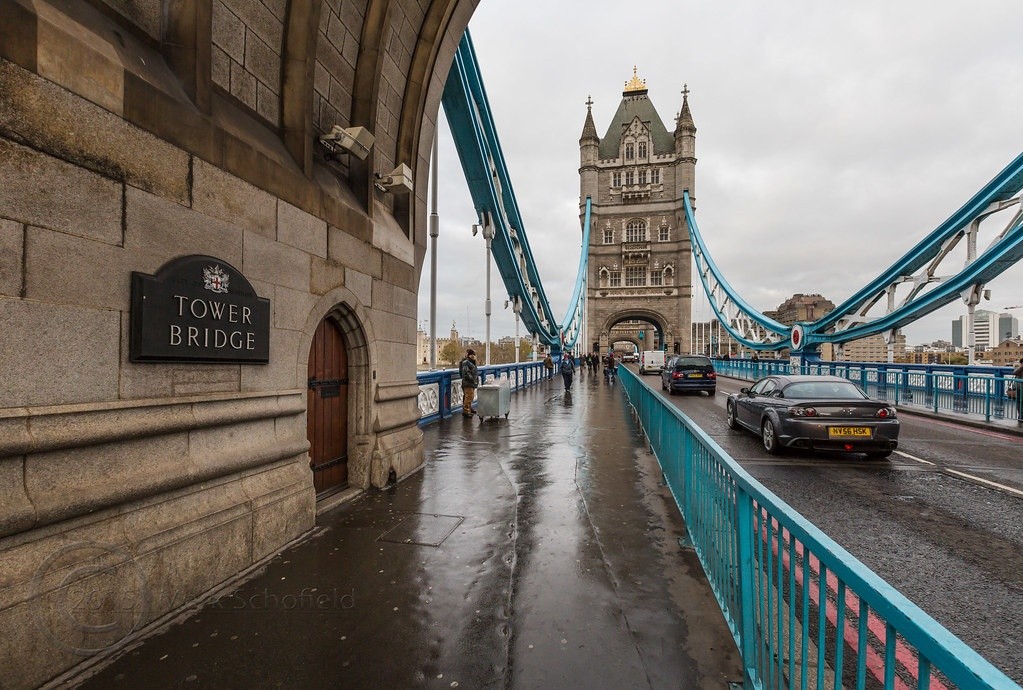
[1007,388,1019,399]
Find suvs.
[660,354,716,397]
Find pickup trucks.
[622,352,635,364]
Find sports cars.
[727,374,900,460]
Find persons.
[544,353,553,379]
[607,353,615,369]
[715,352,730,375]
[561,352,576,392]
[459,349,478,418]
[1013,358,1023,422]
[579,351,600,375]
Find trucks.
[639,350,664,375]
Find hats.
[564,354,568,358]
[467,349,475,356]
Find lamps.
[322,124,416,199]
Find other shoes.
[548,377,554,380]
[566,388,570,392]
[462,409,476,418]
